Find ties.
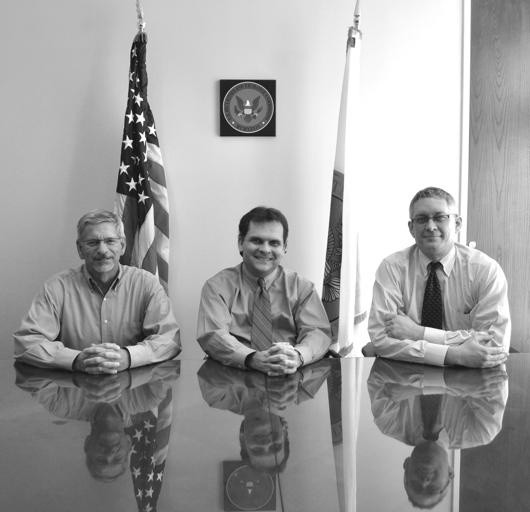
[420,393,443,444]
[421,260,443,330]
[251,277,274,351]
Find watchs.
[298,352,303,364]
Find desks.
[0,352,528,507]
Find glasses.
[411,211,451,224]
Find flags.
[111,30,170,295]
[126,383,179,512]
[321,47,367,359]
[327,359,358,512]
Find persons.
[195,207,332,377]
[366,358,509,509]
[12,360,183,482]
[196,358,332,474]
[11,209,182,376]
[368,186,513,368]
